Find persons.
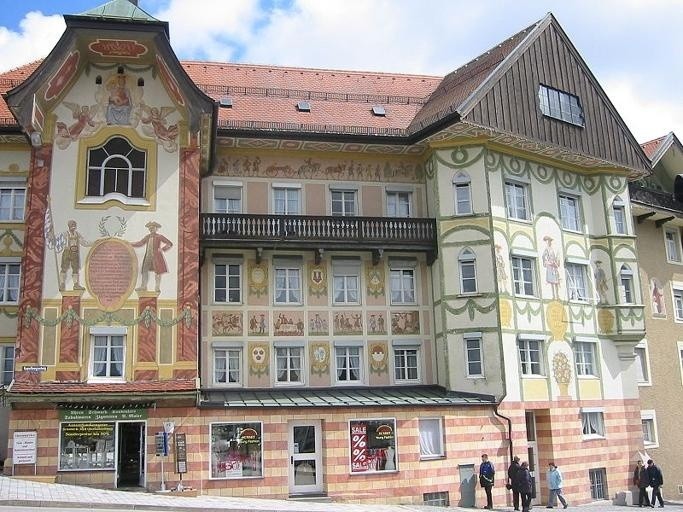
[479,454,494,508]
[652,282,663,313]
[140,104,178,140]
[51,219,89,290]
[541,235,562,300]
[213,312,418,336]
[61,102,98,140]
[505,455,532,509]
[545,462,567,508]
[130,221,172,292]
[516,461,533,512]
[593,260,609,305]
[105,77,133,126]
[647,459,664,507]
[632,459,649,507]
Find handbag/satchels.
[506,483,512,490]
